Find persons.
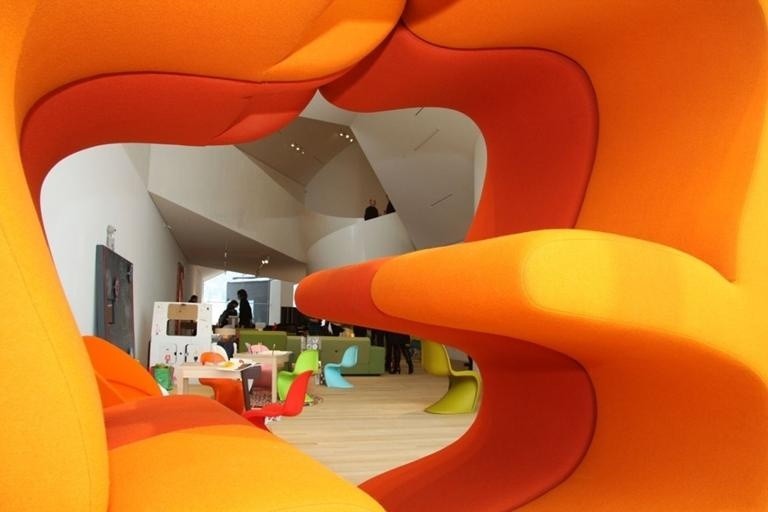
[382,195,395,214]
[295,315,345,336]
[188,295,198,302]
[350,325,414,375]
[218,289,256,330]
[364,196,379,220]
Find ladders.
[174,261,184,335]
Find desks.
[234,346,292,404]
[174,358,255,395]
[238,327,286,352]
[370,344,386,374]
[287,333,371,376]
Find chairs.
[238,370,314,436]
[322,343,359,389]
[417,333,482,415]
[200,350,244,412]
[276,348,319,406]
